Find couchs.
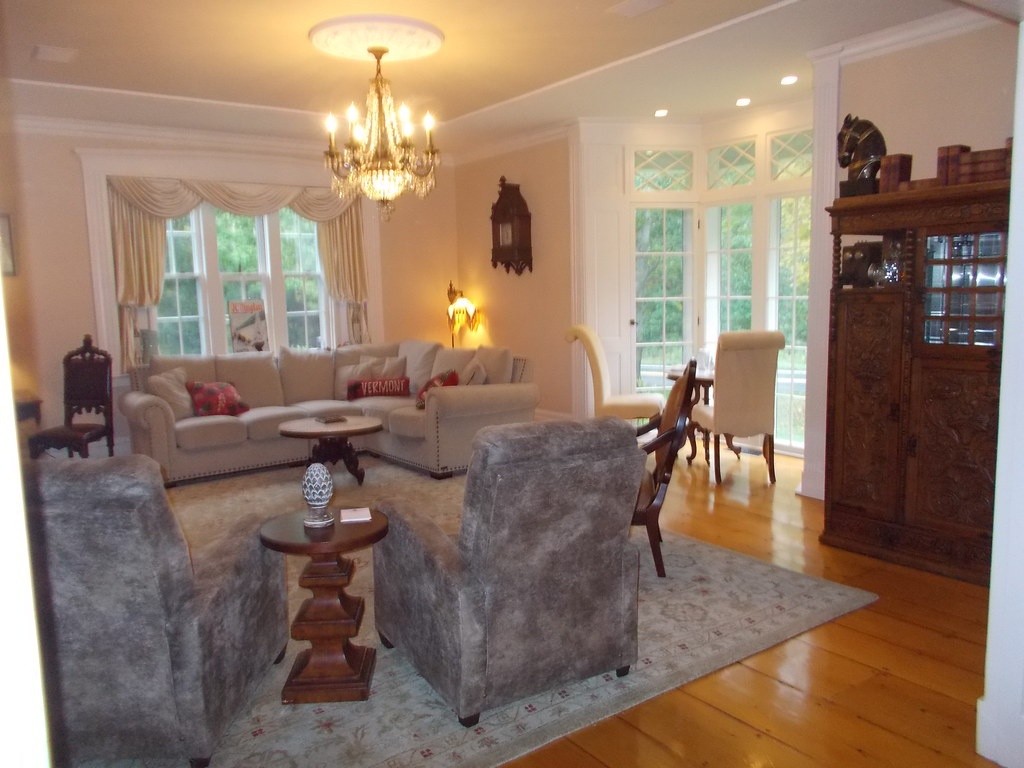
[22,421,648,768]
[118,336,542,489]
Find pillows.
[144,364,250,420]
[333,354,487,410]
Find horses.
[838,114,887,181]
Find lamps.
[307,16,440,223]
[447,280,478,348]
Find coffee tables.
[280,414,383,486]
[259,505,389,706]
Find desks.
[666,374,742,467]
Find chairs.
[27,334,116,459]
[685,328,785,484]
[567,325,666,422]
[630,358,697,577]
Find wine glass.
[867,262,888,288]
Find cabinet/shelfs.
[816,180,1011,587]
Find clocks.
[490,176,533,277]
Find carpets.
[164,447,879,768]
